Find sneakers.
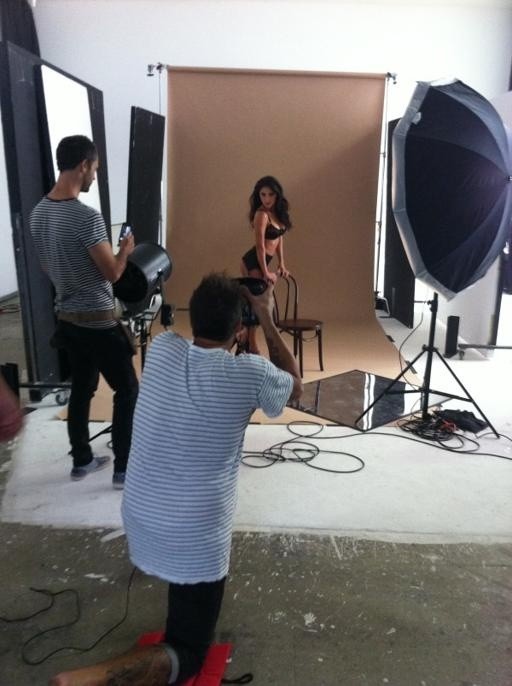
[112,472,126,491]
[70,455,112,481]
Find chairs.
[272,270,322,378]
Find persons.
[230,174,295,356]
[28,134,140,491]
[50,267,306,686]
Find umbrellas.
[389,77,511,421]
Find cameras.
[234,277,268,327]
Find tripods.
[354,292,501,439]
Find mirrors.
[266,369,454,434]
[38,58,100,316]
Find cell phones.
[118,223,136,247]
